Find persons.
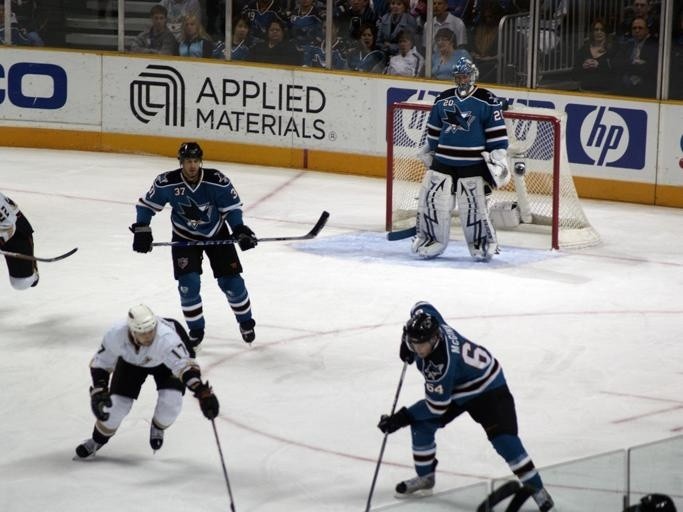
[130,1,682,104]
[131,143,258,350]
[0,1,64,47]
[72,303,219,460]
[377,298,556,511]
[410,55,512,262]
[1,194,39,290]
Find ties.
[631,44,640,85]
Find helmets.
[178,141,203,164]
[128,304,157,333]
[407,313,440,343]
[452,56,480,91]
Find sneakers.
[189,329,204,347]
[76,440,102,457]
[150,423,163,450]
[239,320,256,343]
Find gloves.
[234,225,256,251]
[378,407,410,434]
[194,383,219,419]
[90,383,111,421]
[400,340,416,365]
[132,223,153,254]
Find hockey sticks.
[0,249,79,263]
[387,225,417,239]
[477,480,536,511]
[153,210,329,247]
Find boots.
[396,460,439,493]
[533,488,554,512]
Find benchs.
[65,0,160,52]
